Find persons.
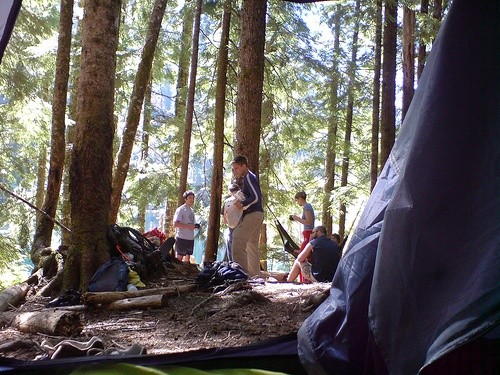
[173,191,202,263]
[284,191,341,283]
[221,155,264,278]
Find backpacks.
[197,259,248,287]
[108,221,161,278]
[86,259,129,293]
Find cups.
[289,214,294,220]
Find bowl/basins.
[194,223,200,228]
